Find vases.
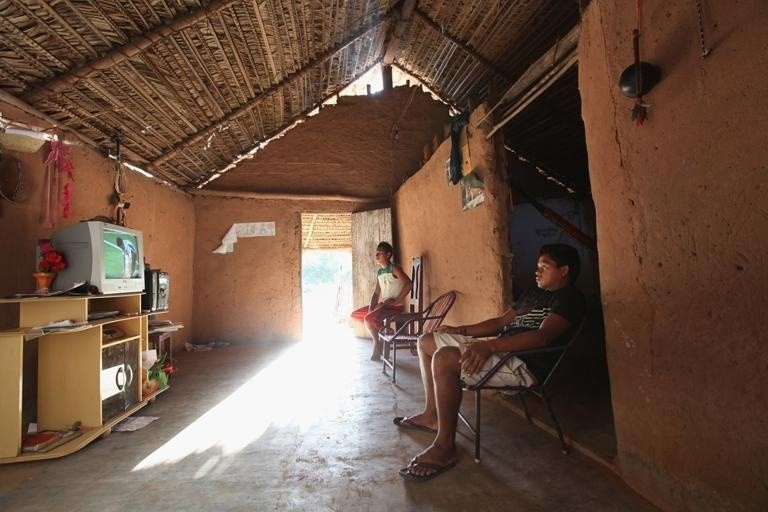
[32,272,58,296]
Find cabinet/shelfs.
[0,289,156,467]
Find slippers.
[394,417,438,434]
[399,457,458,481]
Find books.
[148,318,187,334]
[30,318,93,334]
[21,428,83,453]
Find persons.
[392,242,588,481]
[351,240,414,360]
[115,236,140,279]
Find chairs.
[377,288,454,383]
[462,313,587,465]
[374,255,427,363]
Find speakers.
[142,270,157,311]
[157,270,169,310]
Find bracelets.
[458,326,467,335]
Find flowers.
[33,238,66,273]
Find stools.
[149,330,175,388]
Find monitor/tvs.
[34,221,145,295]
